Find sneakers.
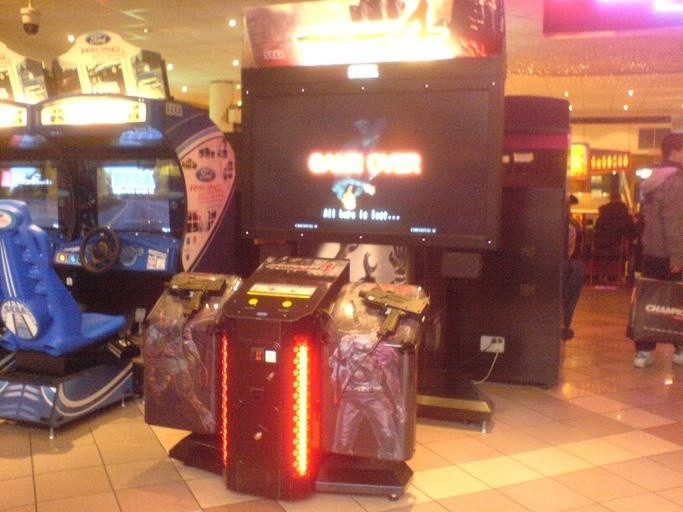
[633,350,654,368]
[670,348,682,366]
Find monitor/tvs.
[0,166,59,229]
[237,56,506,252]
[96,159,171,233]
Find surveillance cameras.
[20,0,40,34]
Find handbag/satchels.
[625,272,681,343]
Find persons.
[633,132,683,368]
[561,192,642,341]
[144,320,217,434]
[328,332,406,460]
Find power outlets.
[480,336,506,354]
